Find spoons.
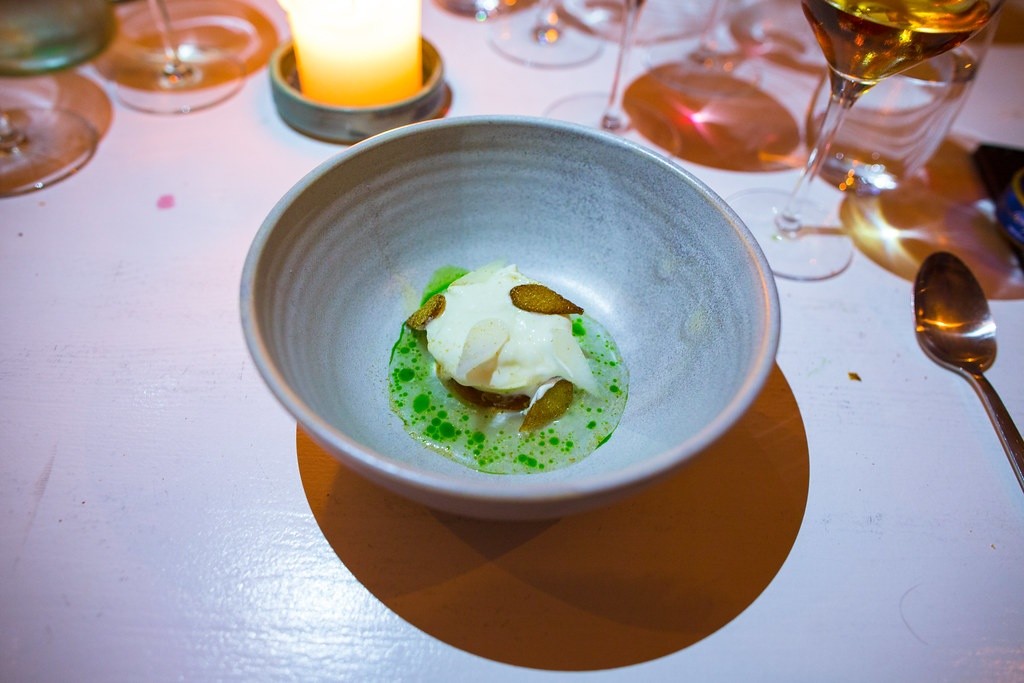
[914,252,1024,492]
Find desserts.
[387,260,627,474]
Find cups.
[805,0,1007,193]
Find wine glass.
[488,0,601,67]
[114,0,246,117]
[552,0,681,162]
[724,0,1004,279]
[0,105,97,198]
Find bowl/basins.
[238,116,782,524]
